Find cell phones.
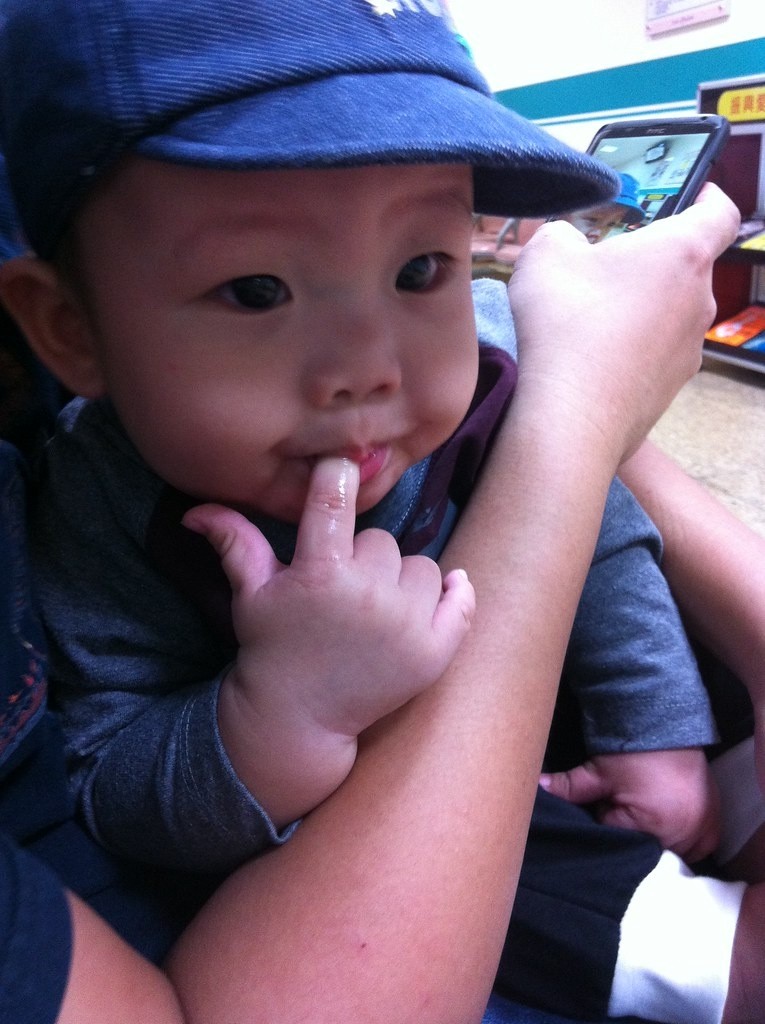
[545,115,732,245]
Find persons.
[557,171,645,246]
[0,0,765,1024]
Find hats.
[610,173,646,224]
[0,0,621,248]
